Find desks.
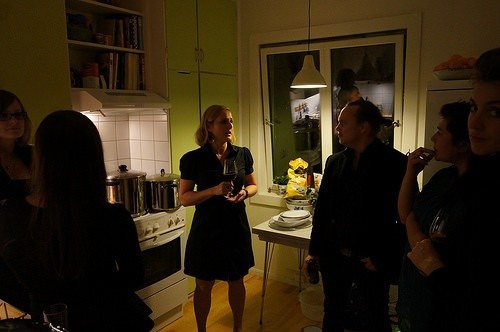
[251,218,314,325]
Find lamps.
[290,0,328,88]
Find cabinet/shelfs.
[65,0,149,95]
[128,0,241,300]
[0,0,73,145]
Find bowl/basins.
[285,198,313,212]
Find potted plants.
[272,175,289,194]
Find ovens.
[131,228,187,332]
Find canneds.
[308,259,319,284]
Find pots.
[106,165,147,217]
[143,169,180,211]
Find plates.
[268,210,313,231]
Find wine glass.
[222,159,238,198]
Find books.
[81,51,140,90]
[97,15,143,50]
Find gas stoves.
[132,205,186,242]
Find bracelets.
[244,189,248,200]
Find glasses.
[0,112,27,121]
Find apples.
[434,54,476,70]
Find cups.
[43,304,68,332]
[428,208,454,244]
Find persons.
[302,99,419,332]
[0,89,41,245]
[409,50,500,332]
[336,68,394,141]
[394,99,474,332]
[0,109,155,332]
[178,104,258,332]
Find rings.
[225,185,230,190]
